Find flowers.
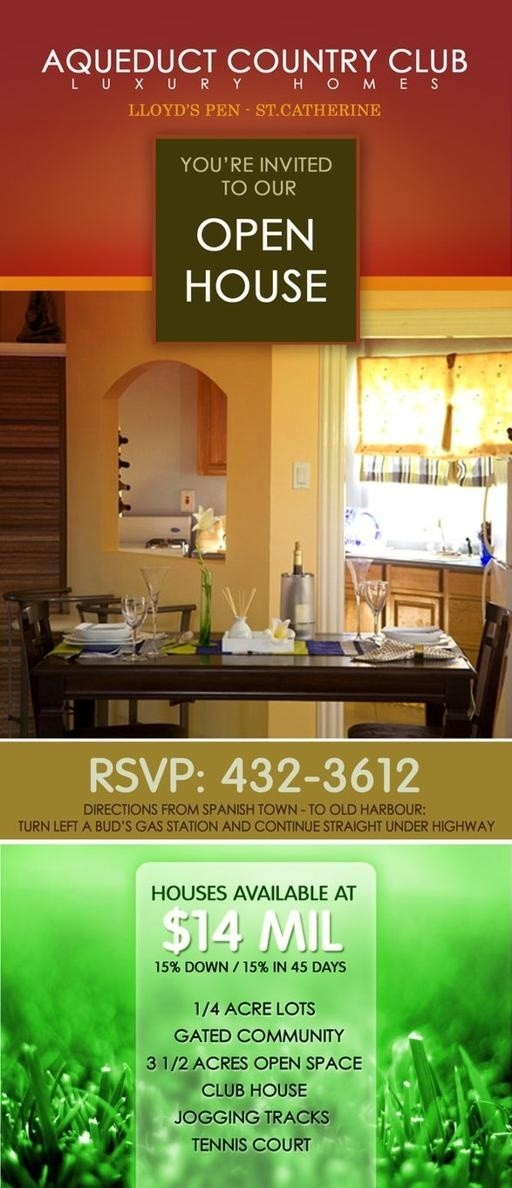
[191,505,220,638]
[265,617,295,645]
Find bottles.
[118,432,133,515]
[280,541,317,639]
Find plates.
[63,621,144,646]
[381,628,443,646]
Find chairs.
[341,602,511,738]
[2,577,195,737]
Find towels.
[349,639,455,662]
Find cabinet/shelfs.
[445,570,488,668]
[340,563,386,630]
[386,560,443,628]
[197,365,228,476]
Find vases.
[192,570,218,645]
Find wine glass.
[344,557,373,643]
[137,565,168,657]
[365,581,391,643]
[120,594,151,662]
